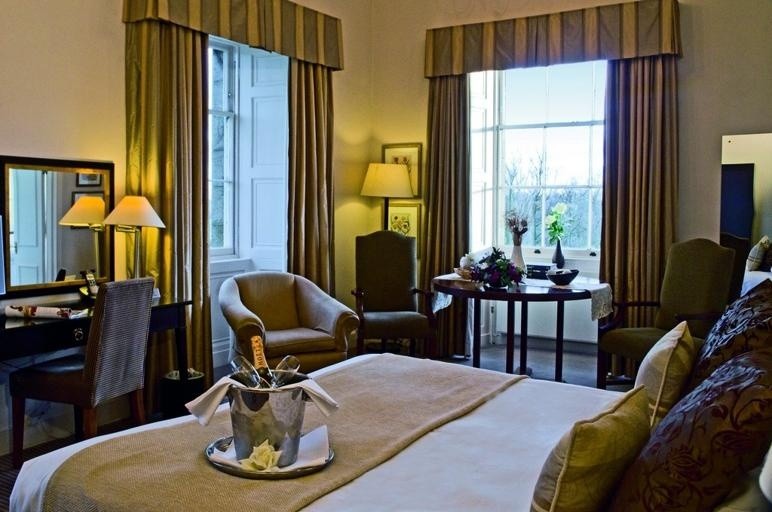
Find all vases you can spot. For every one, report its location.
[551,240,568,267]
[509,243,529,278]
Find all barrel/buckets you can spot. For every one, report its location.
[230,368,309,466]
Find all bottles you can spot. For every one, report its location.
[249,335,273,390]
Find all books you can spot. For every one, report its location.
[2,303,91,320]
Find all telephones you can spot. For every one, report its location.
[79,273,100,305]
[56,268,66,282]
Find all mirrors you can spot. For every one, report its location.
[0,155,118,300]
[720,131,772,273]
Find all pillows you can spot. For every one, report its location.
[619,347,771,512]
[692,276,772,385]
[530,382,652,512]
[631,316,697,416]
[746,229,771,270]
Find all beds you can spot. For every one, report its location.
[6,274,771,512]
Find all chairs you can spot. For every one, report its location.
[217,268,361,386]
[721,230,754,274]
[595,232,738,393]
[349,232,433,358]
[8,275,154,471]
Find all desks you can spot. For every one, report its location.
[425,264,612,382]
[0,294,194,418]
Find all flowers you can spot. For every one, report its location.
[542,201,572,243]
[504,207,533,244]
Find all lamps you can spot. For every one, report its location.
[102,192,168,277]
[361,160,415,232]
[57,195,109,279]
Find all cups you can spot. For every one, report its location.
[218,371,310,466]
[230,355,263,390]
[269,354,301,388]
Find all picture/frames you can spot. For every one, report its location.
[381,142,423,201]
[383,200,422,261]
[75,173,102,187]
[70,190,105,230]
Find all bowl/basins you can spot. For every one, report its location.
[547,270,580,285]
[453,267,473,279]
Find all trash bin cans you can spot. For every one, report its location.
[163,371,205,419]
